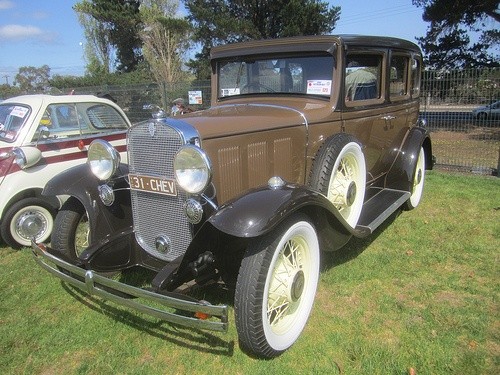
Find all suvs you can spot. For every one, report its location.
[32,34,437,359]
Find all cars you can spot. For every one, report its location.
[473,98,500,121]
[0,94,133,250]
